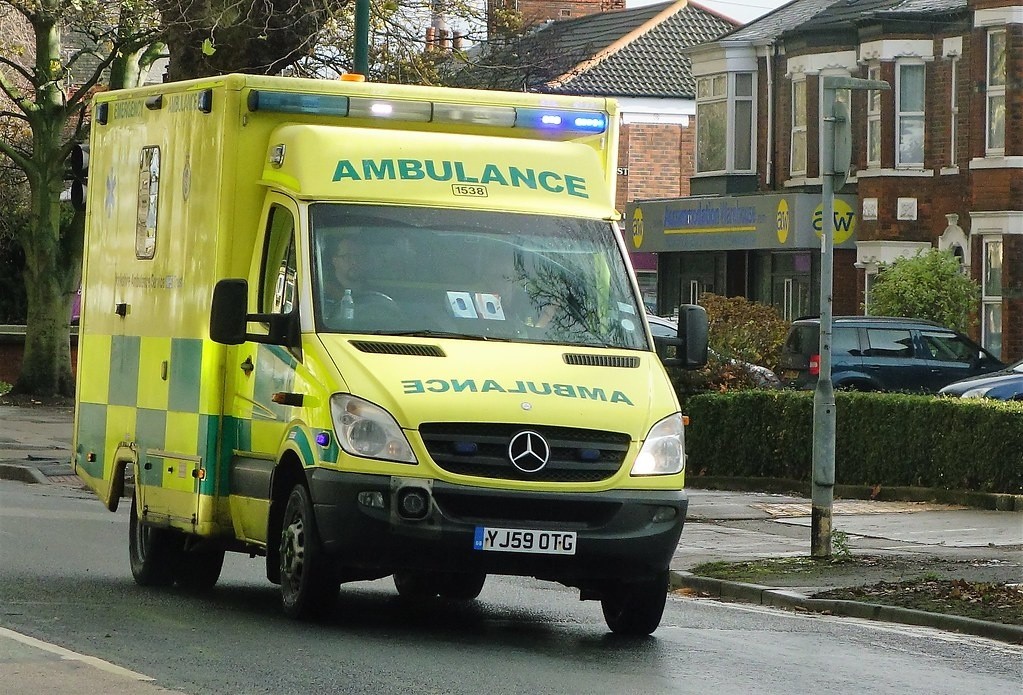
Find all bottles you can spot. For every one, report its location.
[341,289,354,318]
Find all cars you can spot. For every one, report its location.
[938,356,1023,404]
[614,302,780,389]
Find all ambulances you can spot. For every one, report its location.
[68,72,718,630]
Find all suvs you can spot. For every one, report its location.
[780,315,1011,395]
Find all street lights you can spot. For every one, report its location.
[809,72,896,558]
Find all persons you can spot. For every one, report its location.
[479,247,563,328]
[320,235,373,304]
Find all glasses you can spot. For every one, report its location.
[335,254,367,262]
